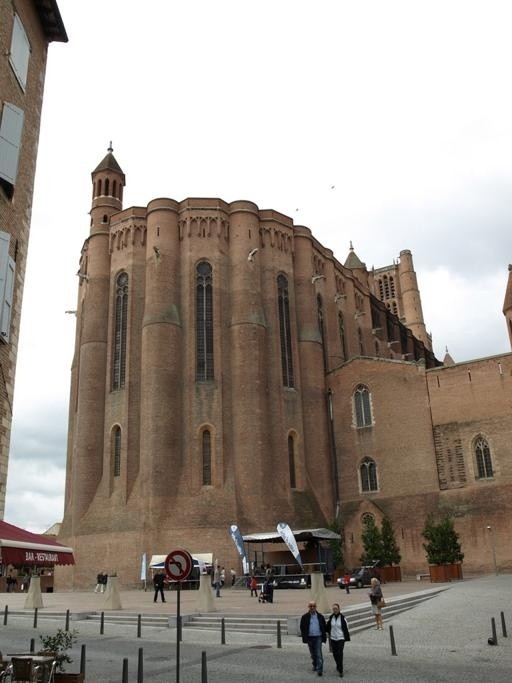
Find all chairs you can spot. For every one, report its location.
[10,656,40,683]
[54,643,86,683]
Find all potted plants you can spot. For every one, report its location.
[420,508,452,583]
[358,514,386,583]
[38,626,81,673]
[440,510,465,582]
[381,515,402,583]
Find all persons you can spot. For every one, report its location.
[326,604,351,677]
[214,560,273,602]
[94,570,104,592]
[344,572,351,594]
[300,602,327,676]
[368,578,384,630]
[8,568,16,591]
[103,572,108,591]
[23,573,30,593]
[153,569,166,603]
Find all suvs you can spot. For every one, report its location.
[337,566,381,588]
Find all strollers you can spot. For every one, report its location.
[259,581,270,601]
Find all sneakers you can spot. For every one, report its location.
[312,666,343,677]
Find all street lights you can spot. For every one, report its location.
[484,522,500,575]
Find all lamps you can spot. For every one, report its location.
[388,340,399,349]
[402,352,413,360]
[153,246,161,260]
[312,275,326,284]
[334,295,347,303]
[64,310,78,317]
[78,272,89,283]
[248,248,258,262]
[354,312,367,321]
[372,328,383,335]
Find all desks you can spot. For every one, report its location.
[167,579,200,591]
[3,654,55,683]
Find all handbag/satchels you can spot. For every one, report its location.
[376,600,387,608]
